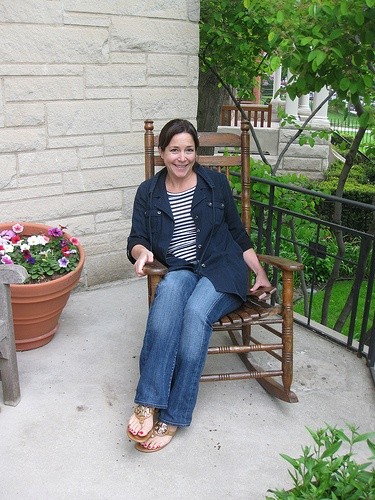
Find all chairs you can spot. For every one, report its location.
[0,264,29,408]
[143,118,304,404]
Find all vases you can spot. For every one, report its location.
[0,221,85,352]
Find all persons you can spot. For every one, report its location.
[127,119,271,453]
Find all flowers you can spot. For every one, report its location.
[0,224,81,278]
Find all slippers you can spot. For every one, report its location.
[136,420,177,453]
[127,404,160,442]
